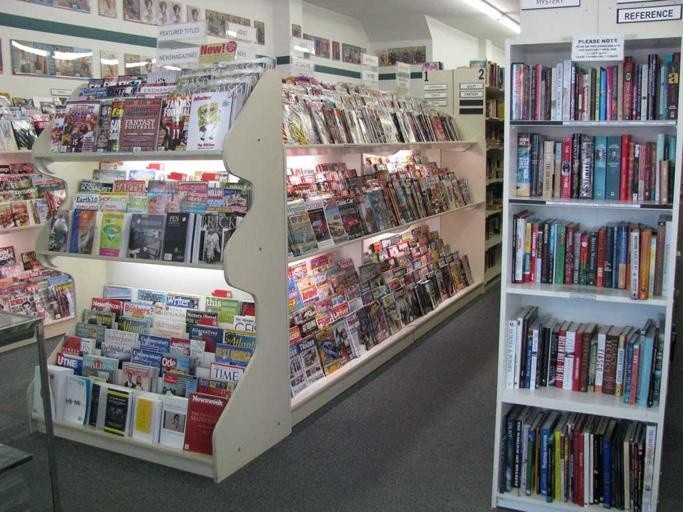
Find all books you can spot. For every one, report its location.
[501,54,682,122]
[503,304,666,408]
[504,405,657,512]
[517,132,676,206]
[1,46,503,456]
[511,210,672,301]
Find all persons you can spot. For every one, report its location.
[144,0,152,21]
[171,5,183,26]
[189,8,201,23]
[156,1,167,24]
